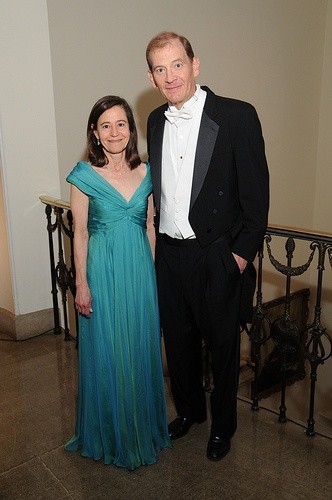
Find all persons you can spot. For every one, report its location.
[64,96,174,470]
[146,31,270,462]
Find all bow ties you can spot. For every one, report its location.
[164,111,192,124]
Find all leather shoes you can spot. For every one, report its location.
[168,415,208,440]
[207,431,230,461]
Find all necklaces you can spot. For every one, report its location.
[105,165,130,176]
